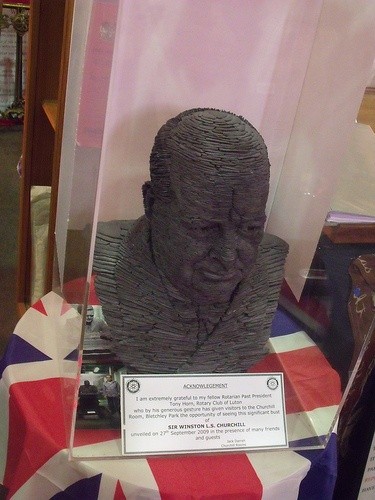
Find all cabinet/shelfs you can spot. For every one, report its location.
[14,0,76,321]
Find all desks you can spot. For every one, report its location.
[6,273,343,500]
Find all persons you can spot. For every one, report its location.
[91,102,287,377]
